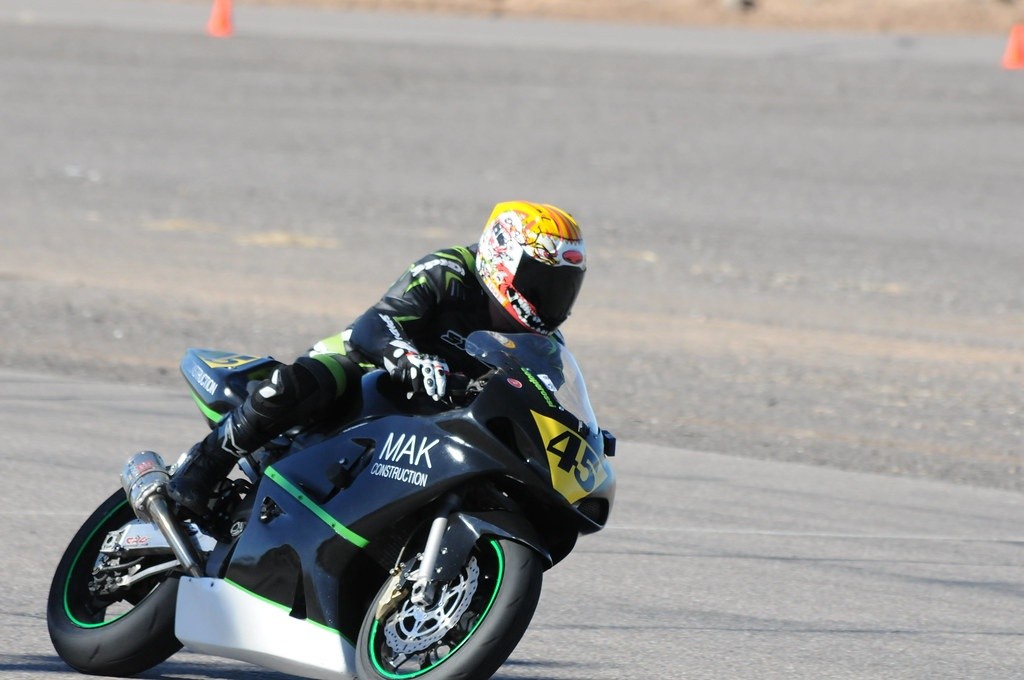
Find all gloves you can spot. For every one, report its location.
[383,340,449,401]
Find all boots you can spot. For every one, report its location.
[167,396,295,516]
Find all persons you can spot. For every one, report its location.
[163,200,590,515]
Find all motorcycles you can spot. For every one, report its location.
[46,333,622,675]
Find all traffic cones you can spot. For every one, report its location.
[1000,18,1024,73]
[204,1,235,37]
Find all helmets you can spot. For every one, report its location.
[474,199,587,338]
